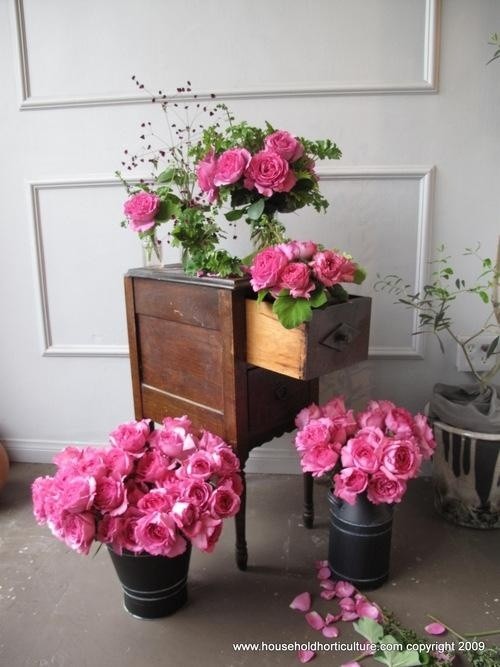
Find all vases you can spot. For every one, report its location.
[109,545,194,620]
[328,482,395,589]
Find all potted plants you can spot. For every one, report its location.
[376,243,498,530]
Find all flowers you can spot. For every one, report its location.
[108,76,368,330]
[292,394,433,507]
[29,413,244,559]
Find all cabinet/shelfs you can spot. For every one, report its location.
[125,265,372,574]
